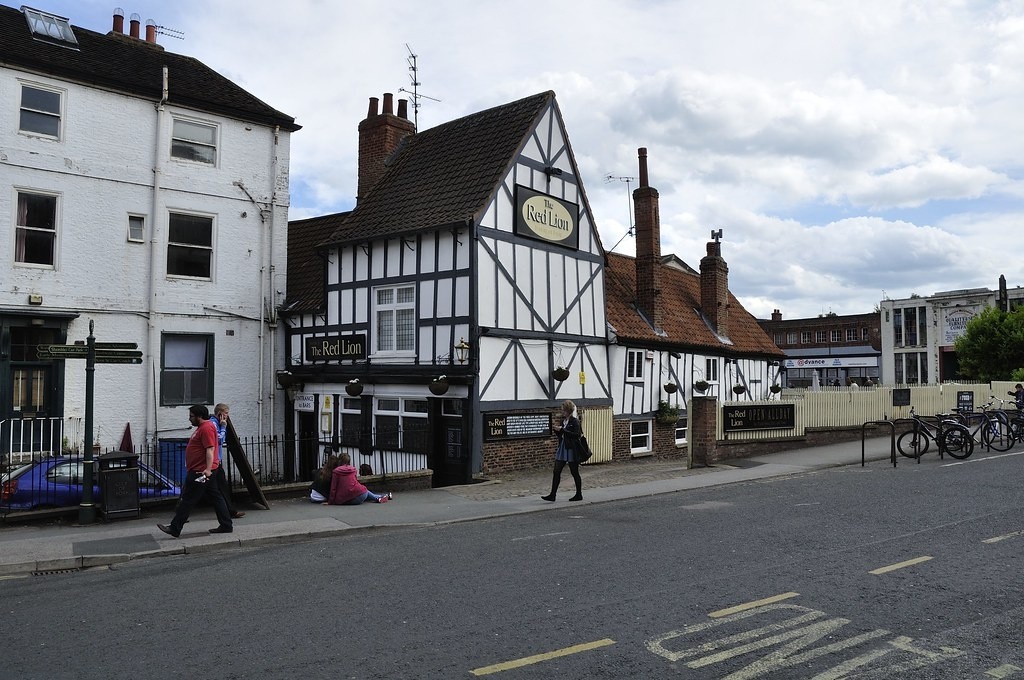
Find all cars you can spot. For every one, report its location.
[0,453,182,512]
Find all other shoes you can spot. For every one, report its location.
[157,524,179,537]
[569,495,582,501]
[232,512,245,518]
[389,493,392,500]
[209,525,233,533]
[379,496,388,503]
[541,494,555,501]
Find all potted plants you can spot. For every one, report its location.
[551,366,571,382]
[655,399,681,425]
[696,380,709,391]
[733,383,746,394]
[93,442,101,455]
[770,384,782,394]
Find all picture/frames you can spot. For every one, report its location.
[321,412,332,434]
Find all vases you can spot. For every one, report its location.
[345,385,363,397]
[428,383,450,396]
[278,375,293,384]
[664,385,678,394]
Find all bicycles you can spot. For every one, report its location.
[938,401,1015,452]
[897,405,974,460]
[989,395,1024,444]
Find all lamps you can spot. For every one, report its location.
[454,337,470,367]
[670,352,681,360]
[725,358,738,365]
[31,319,45,329]
[28,294,43,306]
[545,165,563,183]
[477,327,491,339]
[768,361,779,366]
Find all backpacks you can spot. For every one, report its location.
[570,418,592,462]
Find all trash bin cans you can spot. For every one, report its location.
[96,451,143,522]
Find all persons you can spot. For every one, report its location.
[541,399,583,502]
[849,379,859,387]
[1008,384,1024,409]
[320,453,393,505]
[834,379,841,386]
[863,376,873,387]
[819,382,824,386]
[306,454,339,504]
[157,405,233,537]
[185,403,246,523]
[788,384,795,388]
[845,377,852,386]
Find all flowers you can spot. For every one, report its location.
[664,380,676,386]
[431,375,448,384]
[348,378,361,386]
[276,371,293,376]
